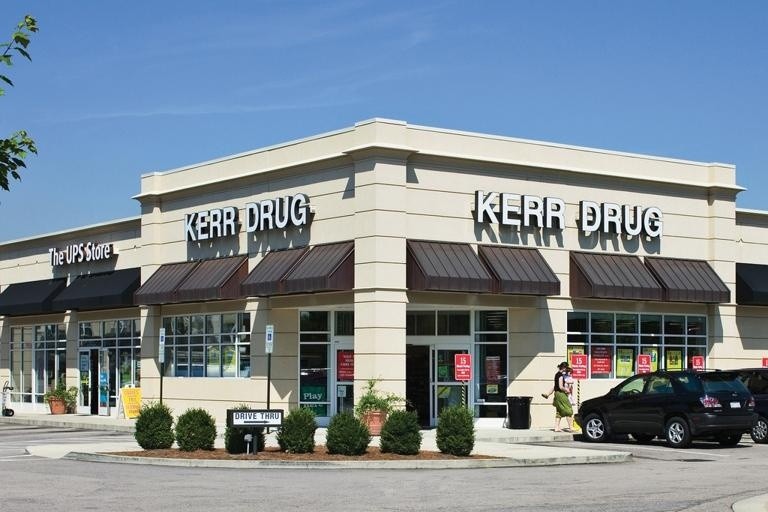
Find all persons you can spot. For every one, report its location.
[541,367,577,402]
[552,360,577,433]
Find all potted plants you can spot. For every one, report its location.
[353,379,414,436]
[44,378,77,414]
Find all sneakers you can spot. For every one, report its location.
[542,392,549,399]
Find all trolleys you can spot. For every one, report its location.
[1,380,14,417]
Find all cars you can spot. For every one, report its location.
[578,369,768,448]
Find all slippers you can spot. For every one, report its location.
[554,428,562,432]
[568,428,576,433]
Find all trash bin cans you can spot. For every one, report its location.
[505,396,533,429]
